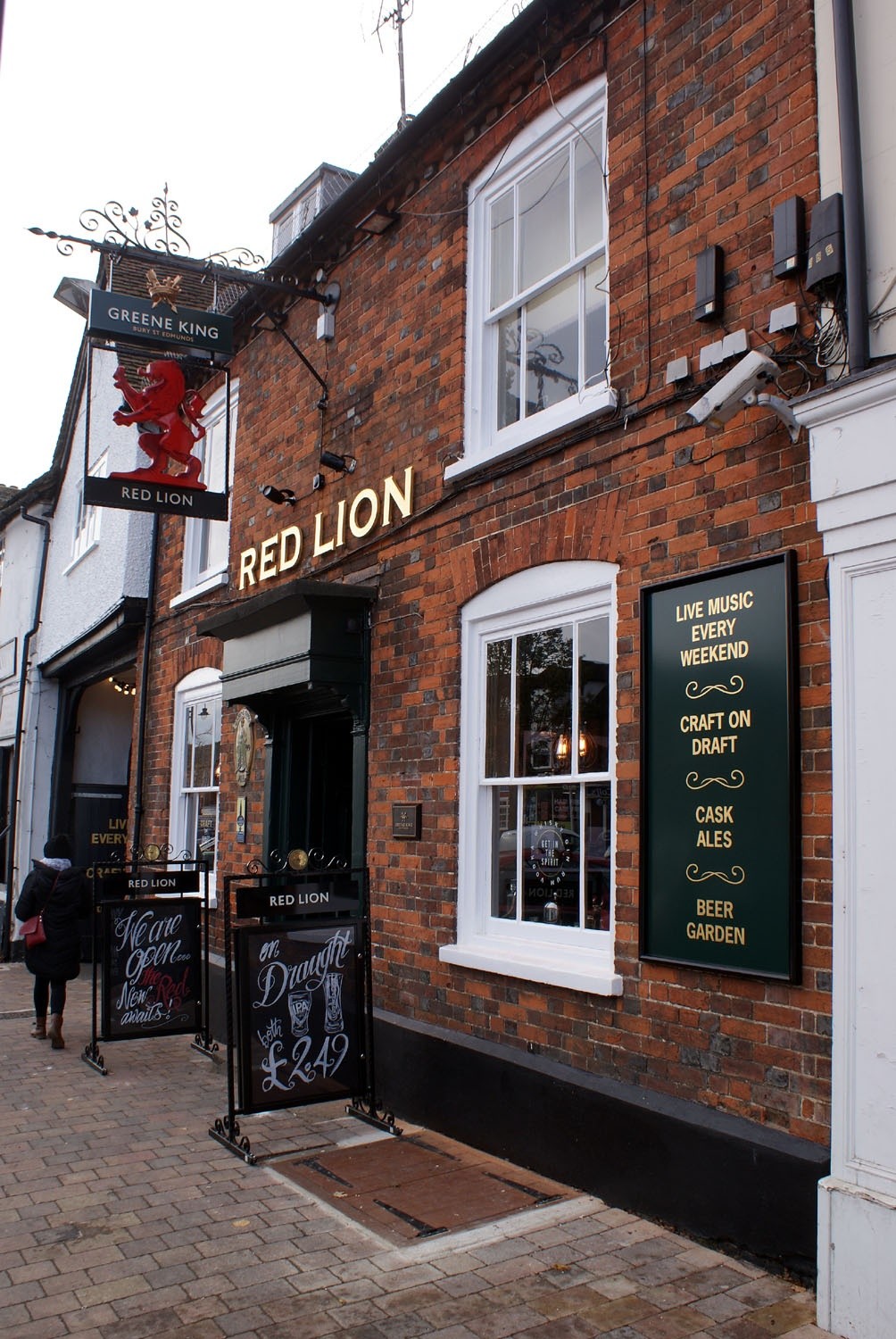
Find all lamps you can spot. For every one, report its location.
[320,452,356,473]
[109,676,137,697]
[355,209,399,235]
[263,485,296,507]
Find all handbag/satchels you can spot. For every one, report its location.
[18,917,46,947]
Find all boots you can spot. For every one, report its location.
[47,1014,66,1049]
[31,1018,47,1039]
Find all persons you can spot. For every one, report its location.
[15,834,87,1049]
